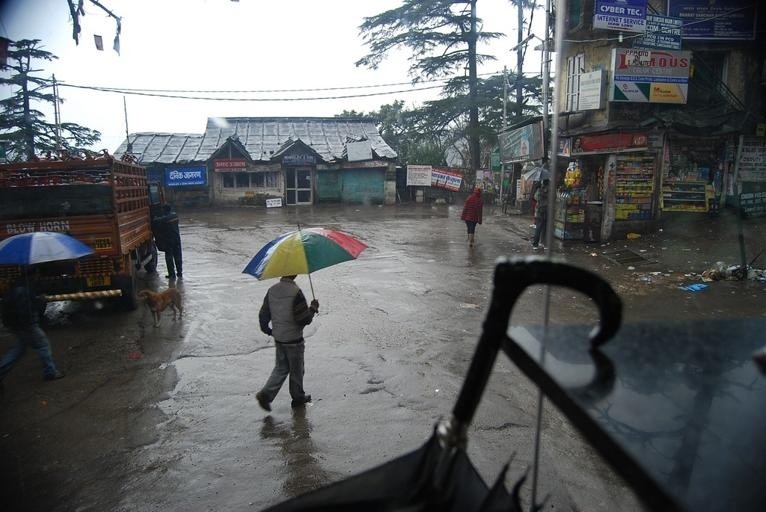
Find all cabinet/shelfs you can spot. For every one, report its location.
[552,183,587,241]
[595,152,722,220]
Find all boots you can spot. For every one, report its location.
[469,234,475,248]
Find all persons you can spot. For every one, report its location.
[461,187,484,248]
[529,179,550,251]
[254,276,319,412]
[0,265,66,381]
[572,137,584,153]
[152,204,182,278]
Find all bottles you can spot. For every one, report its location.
[616,191,632,203]
[559,192,573,206]
[683,176,687,182]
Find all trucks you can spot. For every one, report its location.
[0,148,156,315]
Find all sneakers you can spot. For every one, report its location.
[177,272,182,277]
[44,370,64,379]
[532,247,538,250]
[256,391,272,411]
[165,274,176,278]
[291,394,311,409]
[529,224,536,229]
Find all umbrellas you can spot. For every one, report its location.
[0,231,96,266]
[241,219,369,313]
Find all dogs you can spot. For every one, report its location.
[136,288,184,328]
